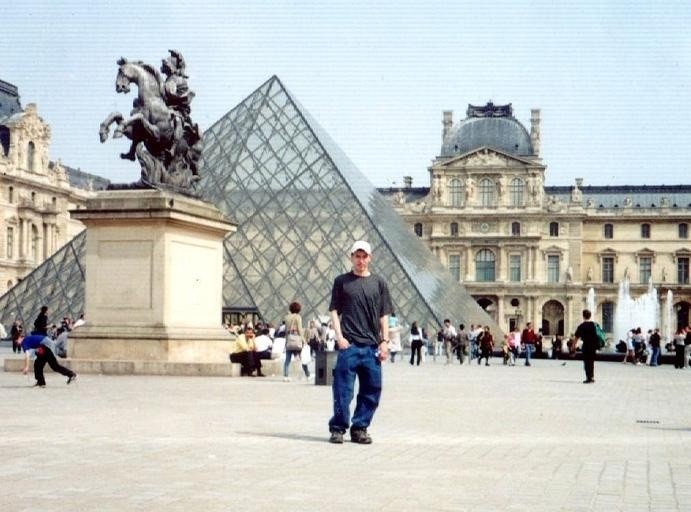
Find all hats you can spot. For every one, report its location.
[351,240,372,257]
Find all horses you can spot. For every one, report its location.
[97,56,202,180]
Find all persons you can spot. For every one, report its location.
[328,240,396,444]
[120,50,191,161]
[10,305,85,389]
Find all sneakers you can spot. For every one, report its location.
[33,384,46,389]
[350,426,371,444]
[329,431,344,443]
[67,373,78,384]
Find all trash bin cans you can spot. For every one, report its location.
[315,351,338,386]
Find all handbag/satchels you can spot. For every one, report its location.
[286,333,304,352]
[593,321,604,348]
